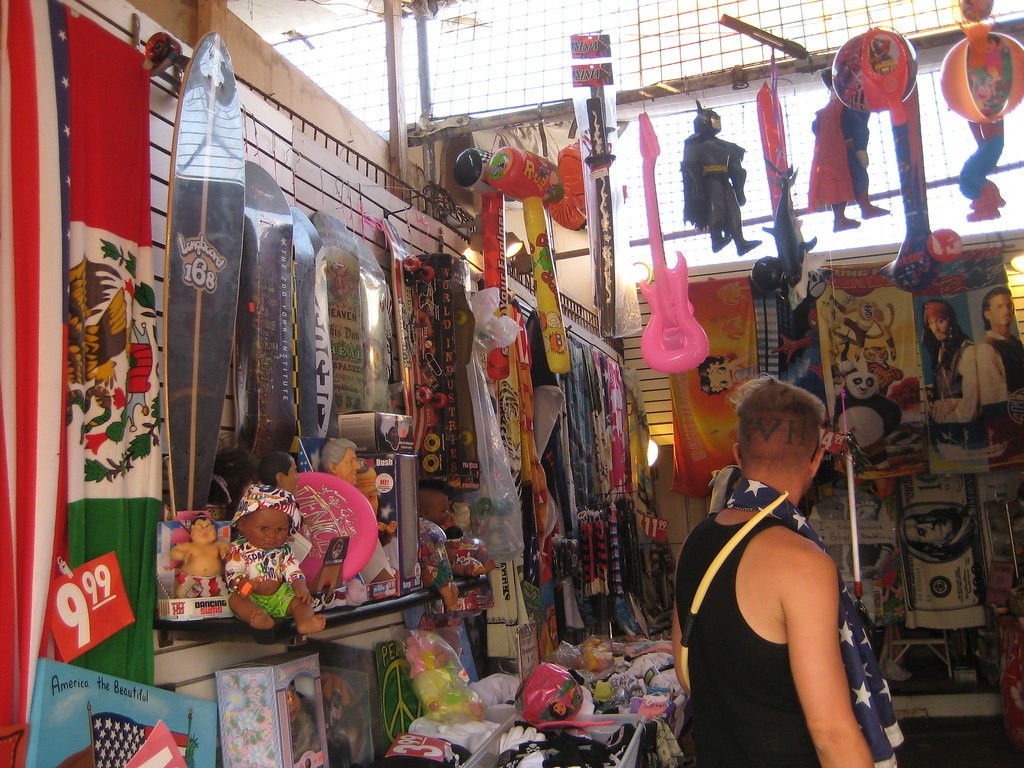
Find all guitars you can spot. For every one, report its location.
[637,110,710,376]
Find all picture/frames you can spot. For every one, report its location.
[287,638,385,768]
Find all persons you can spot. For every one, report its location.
[670,382,875,768]
[923,287,1024,446]
[170,437,360,634]
[417,487,495,612]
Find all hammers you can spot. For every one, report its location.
[456,144,573,378]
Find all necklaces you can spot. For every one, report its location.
[734,506,757,512]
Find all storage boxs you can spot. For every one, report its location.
[312,577,398,613]
[215,649,329,768]
[454,708,645,768]
[337,410,416,456]
[156,596,232,621]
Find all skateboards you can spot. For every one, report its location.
[230,158,393,474]
[382,214,449,456]
[138,29,249,523]
